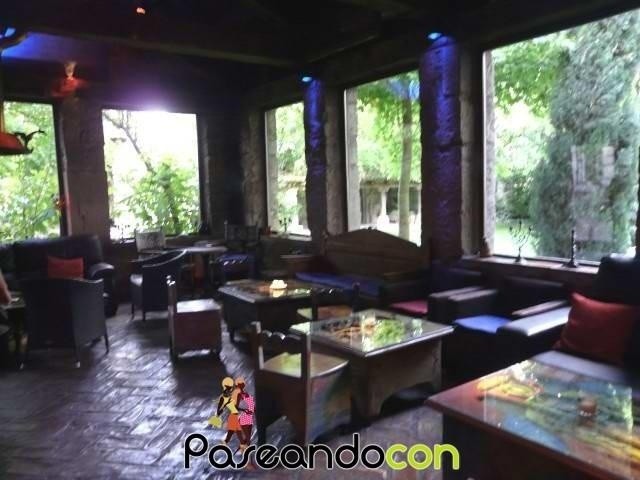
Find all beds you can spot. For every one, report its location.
[420,349,640,480]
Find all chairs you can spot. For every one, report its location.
[18,278,110,372]
[129,220,261,366]
[378,253,640,387]
[250,320,353,459]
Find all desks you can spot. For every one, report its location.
[217,278,454,427]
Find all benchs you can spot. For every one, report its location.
[280,226,432,312]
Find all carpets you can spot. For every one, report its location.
[252,404,444,480]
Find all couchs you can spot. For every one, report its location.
[0,233,121,317]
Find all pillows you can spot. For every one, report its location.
[46,255,85,280]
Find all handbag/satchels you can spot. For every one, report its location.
[239,399,249,410]
[207,415,222,429]
